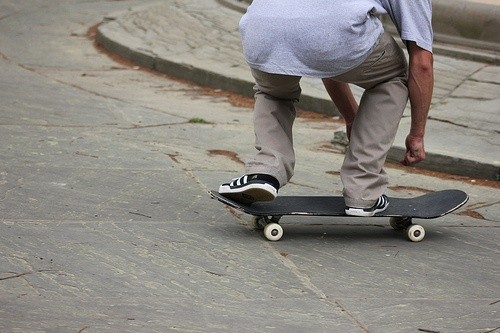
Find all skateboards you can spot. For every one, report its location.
[206,189,470,242]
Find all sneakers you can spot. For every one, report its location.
[218,174,280,207]
[345,194,390,216]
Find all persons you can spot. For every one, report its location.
[219,0,432,215]
